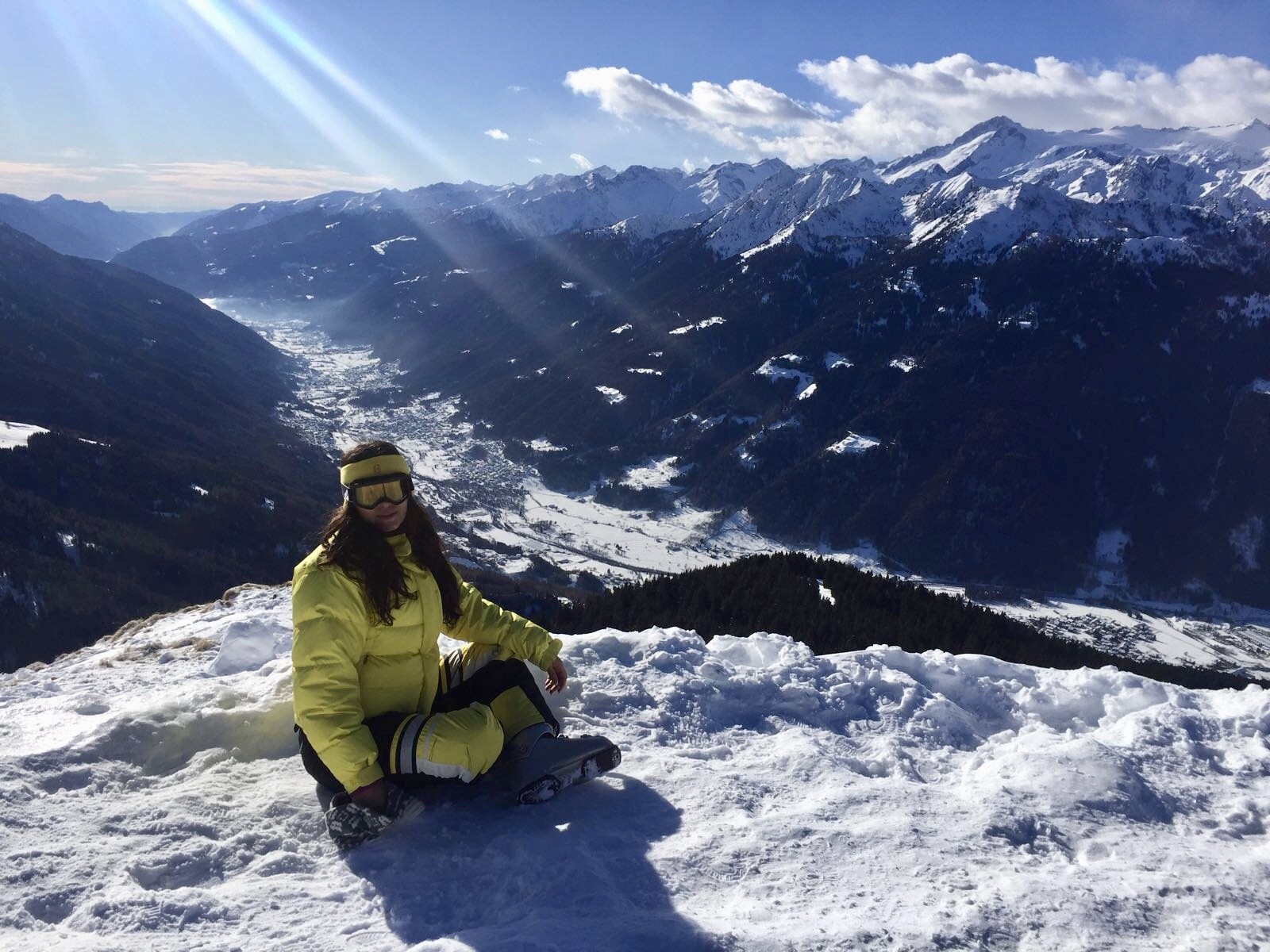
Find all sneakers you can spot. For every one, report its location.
[509,733,621,804]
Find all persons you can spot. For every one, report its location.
[292,440,621,840]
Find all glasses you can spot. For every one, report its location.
[350,474,408,509]
